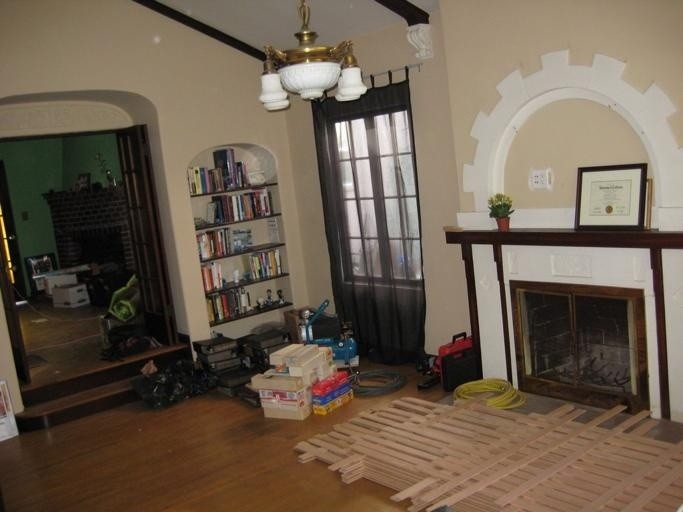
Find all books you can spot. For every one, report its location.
[188,148,282,324]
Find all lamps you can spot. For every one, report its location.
[256,2,368,112]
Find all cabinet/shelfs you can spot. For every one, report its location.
[189,182,293,327]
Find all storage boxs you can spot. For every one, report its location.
[44,273,106,311]
[192,302,363,422]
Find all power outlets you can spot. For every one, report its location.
[532,170,547,190]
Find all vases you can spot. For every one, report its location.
[495,216,511,232]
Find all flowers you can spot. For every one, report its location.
[486,193,517,220]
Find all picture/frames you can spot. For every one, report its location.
[644,178,654,230]
[574,161,648,231]
[78,173,91,194]
[24,252,60,300]
